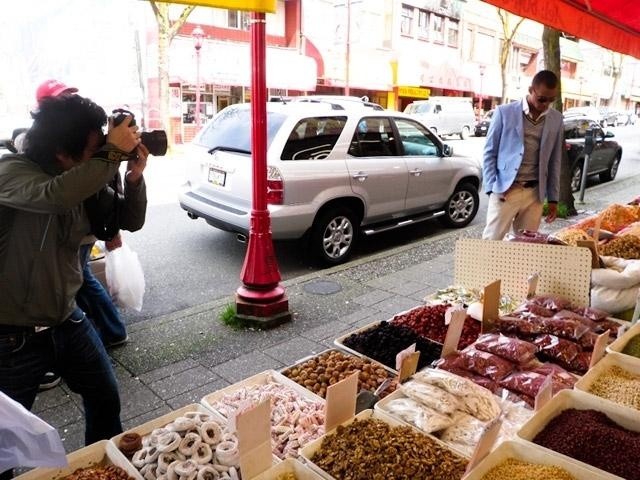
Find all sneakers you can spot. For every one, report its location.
[37,373,60,391]
[99,330,129,348]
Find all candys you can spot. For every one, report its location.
[210,373,326,460]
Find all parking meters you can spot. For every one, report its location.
[579,129,596,207]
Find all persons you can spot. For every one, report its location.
[13,76,131,390]
[481,69,564,241]
[0,93,149,480]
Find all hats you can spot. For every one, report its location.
[36,77,79,103]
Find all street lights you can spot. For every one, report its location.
[626,78,637,110]
[478,61,493,124]
[190,22,204,135]
[578,76,585,108]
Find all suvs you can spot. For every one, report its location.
[176,101,484,267]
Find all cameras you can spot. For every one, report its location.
[109,109,167,160]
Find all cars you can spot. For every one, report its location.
[564,103,638,127]
[1,240,106,355]
[279,92,384,104]
[475,108,496,138]
[560,111,624,191]
[0,91,34,155]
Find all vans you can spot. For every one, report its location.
[399,95,477,139]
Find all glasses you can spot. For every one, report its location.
[533,89,558,103]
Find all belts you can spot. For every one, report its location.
[1,324,49,335]
[523,180,538,188]
